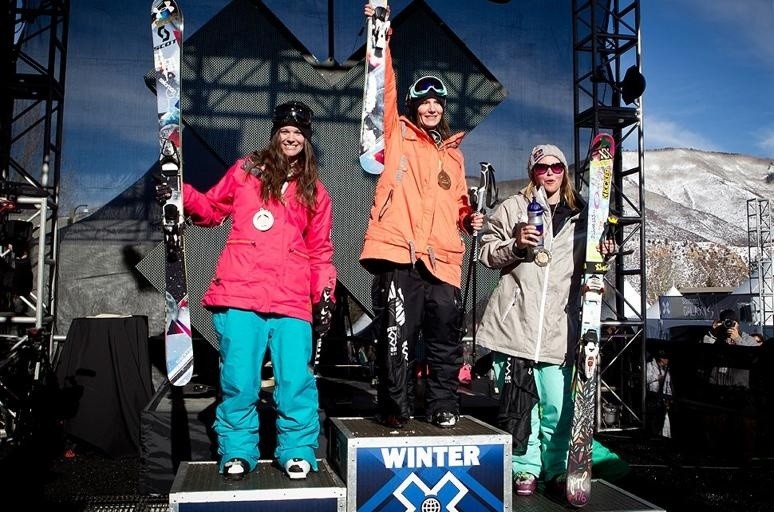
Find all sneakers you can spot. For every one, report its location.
[553,472,567,491]
[513,471,537,496]
[224,458,249,481]
[284,458,310,479]
[385,415,403,427]
[428,408,457,428]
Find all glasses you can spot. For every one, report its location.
[272,104,313,127]
[533,163,563,175]
[405,76,448,107]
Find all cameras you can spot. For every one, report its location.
[722,319,734,328]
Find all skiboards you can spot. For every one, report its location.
[152,0,195,388]
[359,0,388,175]
[567,134,614,508]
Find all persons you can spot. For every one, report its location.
[360,3,483,427]
[155,100,337,480]
[701,307,760,387]
[601,317,635,335]
[646,354,673,438]
[475,143,618,497]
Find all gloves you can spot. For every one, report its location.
[315,300,336,332]
[151,173,172,207]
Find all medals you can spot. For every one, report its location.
[438,170,451,190]
[535,248,552,267]
[252,210,274,231]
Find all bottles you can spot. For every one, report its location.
[527,196,544,250]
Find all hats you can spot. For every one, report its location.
[409,91,445,119]
[269,101,312,144]
[720,309,739,324]
[528,144,569,184]
